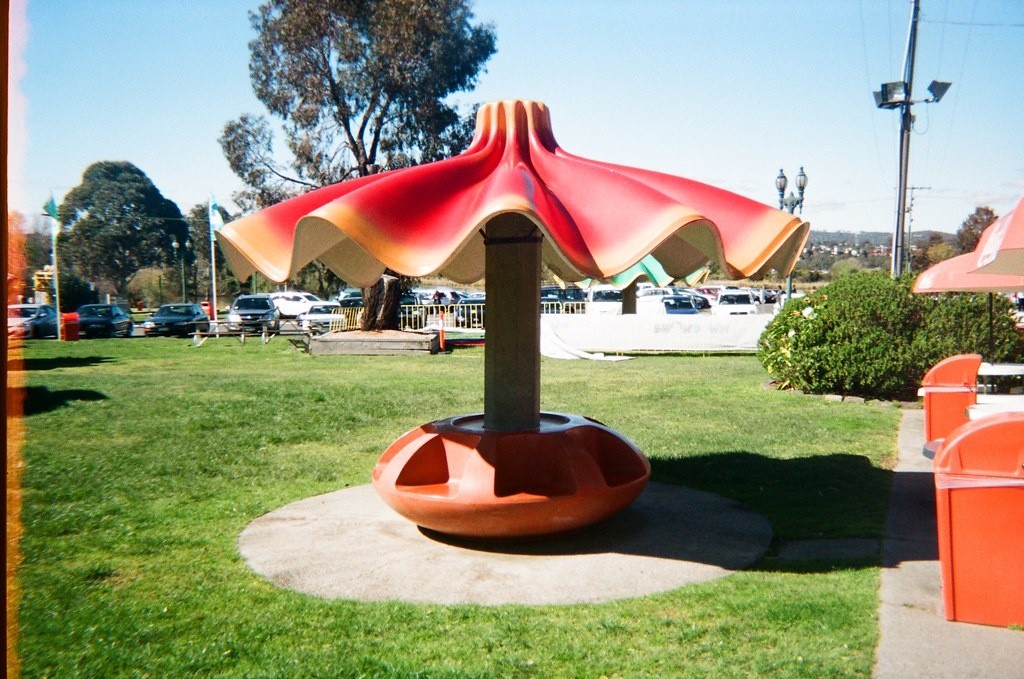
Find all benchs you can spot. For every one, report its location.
[257,319,323,345]
[187,321,251,347]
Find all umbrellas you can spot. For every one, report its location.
[553,253,709,318]
[209,95,811,428]
[913,197,1023,394]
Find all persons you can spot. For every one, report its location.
[759,284,820,304]
[433,291,441,306]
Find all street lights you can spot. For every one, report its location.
[172,239,193,304]
[776,165,809,302]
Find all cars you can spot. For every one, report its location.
[226,294,280,336]
[73,304,134,339]
[336,286,777,314]
[397,295,428,328]
[141,303,210,336]
[6,304,59,339]
[271,291,343,316]
[710,289,757,316]
[663,294,700,315]
[455,298,485,327]
[777,288,808,310]
[299,300,346,335]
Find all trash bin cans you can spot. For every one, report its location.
[916,354,984,442]
[200,301,213,320]
[922,412,1024,629]
